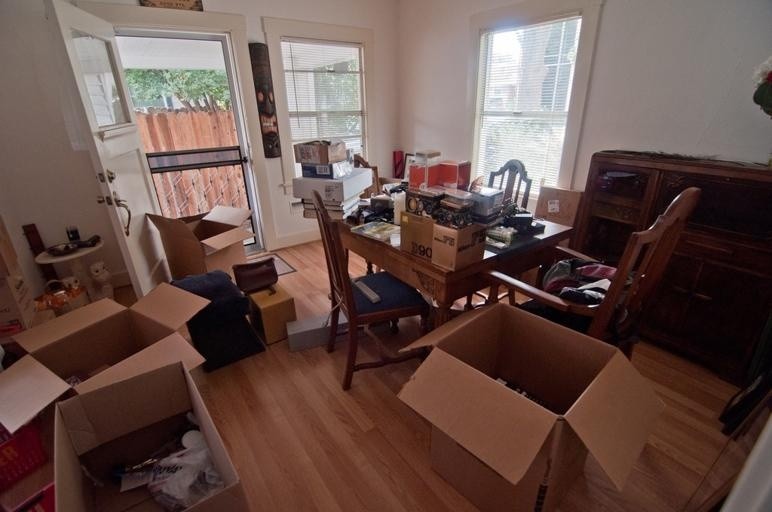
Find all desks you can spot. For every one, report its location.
[35,240,106,304]
[332,205,579,373]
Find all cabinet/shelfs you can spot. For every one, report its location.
[576,152,661,269]
[635,155,770,385]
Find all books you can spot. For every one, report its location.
[350,220,400,242]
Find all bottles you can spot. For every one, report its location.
[394,188,406,225]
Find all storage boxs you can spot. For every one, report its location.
[292,139,503,268]
[1,283,210,434]
[535,185,583,229]
[251,285,297,345]
[0,222,57,367]
[390,301,666,512]
[145,204,256,286]
[54,360,250,511]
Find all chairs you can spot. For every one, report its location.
[489,181,704,369]
[488,156,530,204]
[303,188,431,394]
